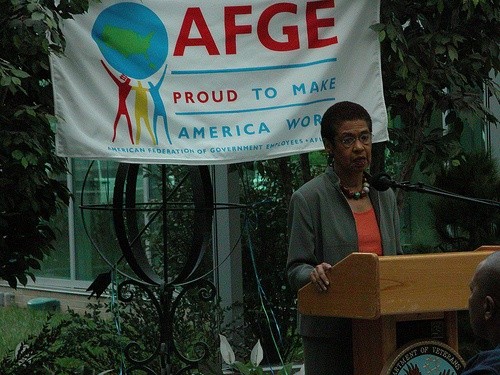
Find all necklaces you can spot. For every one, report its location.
[338,177,377,199]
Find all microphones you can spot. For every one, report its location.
[372,173,407,192]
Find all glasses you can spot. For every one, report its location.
[335,133,374,149]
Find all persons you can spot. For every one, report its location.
[286,101,403,375]
[454,250,500,375]
